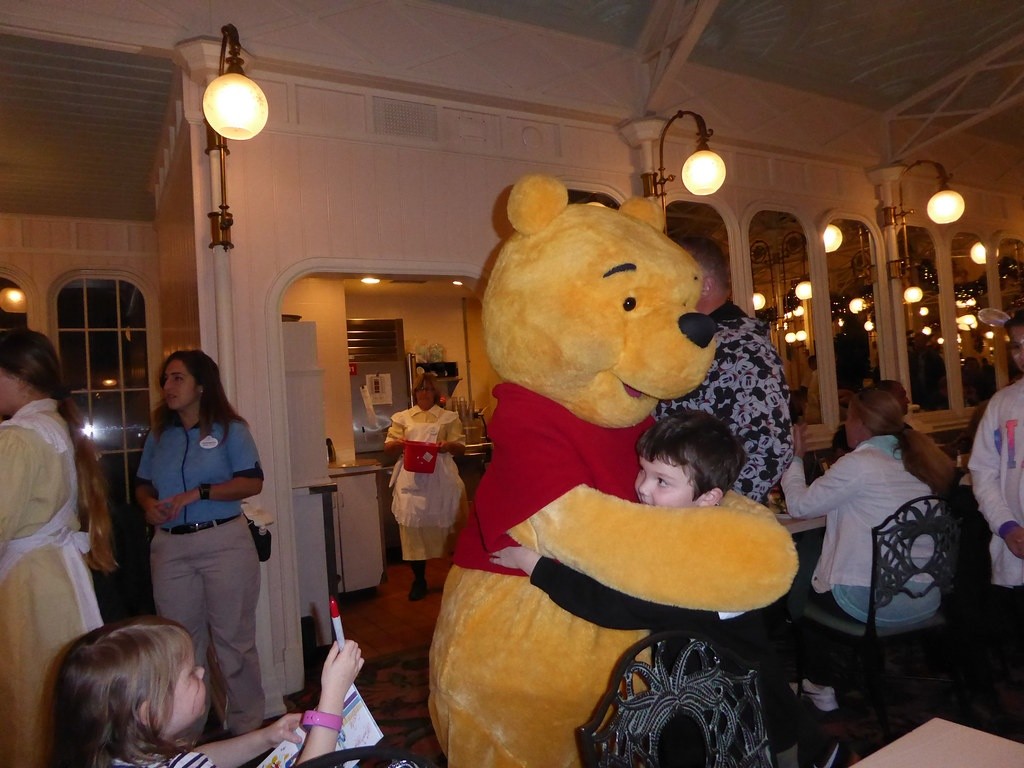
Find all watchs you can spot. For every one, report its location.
[200,482,211,501]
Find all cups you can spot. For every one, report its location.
[452,397,475,418]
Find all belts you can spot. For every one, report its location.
[160,514,241,535]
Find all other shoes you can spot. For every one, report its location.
[409,578,427,601]
[790,679,839,711]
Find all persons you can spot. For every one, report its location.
[383,373,470,600]
[802,331,997,424]
[649,235,795,505]
[49,613,365,768]
[779,379,956,713]
[0,328,123,766]
[136,350,264,737]
[486,411,807,767]
[968,310,1024,699]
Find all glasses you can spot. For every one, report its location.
[419,385,433,391]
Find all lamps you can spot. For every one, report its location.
[0,282,29,321]
[904,152,969,228]
[179,23,276,249]
[639,102,730,201]
[744,220,1016,370]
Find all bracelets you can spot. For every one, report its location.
[197,485,203,500]
[999,521,1019,539]
[302,710,343,734]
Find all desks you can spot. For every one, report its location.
[765,496,826,538]
[840,716,1024,768]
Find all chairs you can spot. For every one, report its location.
[575,624,779,768]
[789,491,966,709]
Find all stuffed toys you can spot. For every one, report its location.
[426,176,800,767]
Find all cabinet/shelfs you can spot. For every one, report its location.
[327,460,387,599]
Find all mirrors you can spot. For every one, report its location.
[735,199,833,444]
[941,222,1005,417]
[885,216,963,421]
[815,208,899,433]
[986,228,1024,390]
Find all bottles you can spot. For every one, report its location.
[259,526,266,535]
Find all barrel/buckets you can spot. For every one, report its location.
[403,441,438,473]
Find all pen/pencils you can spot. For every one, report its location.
[329,596,346,651]
